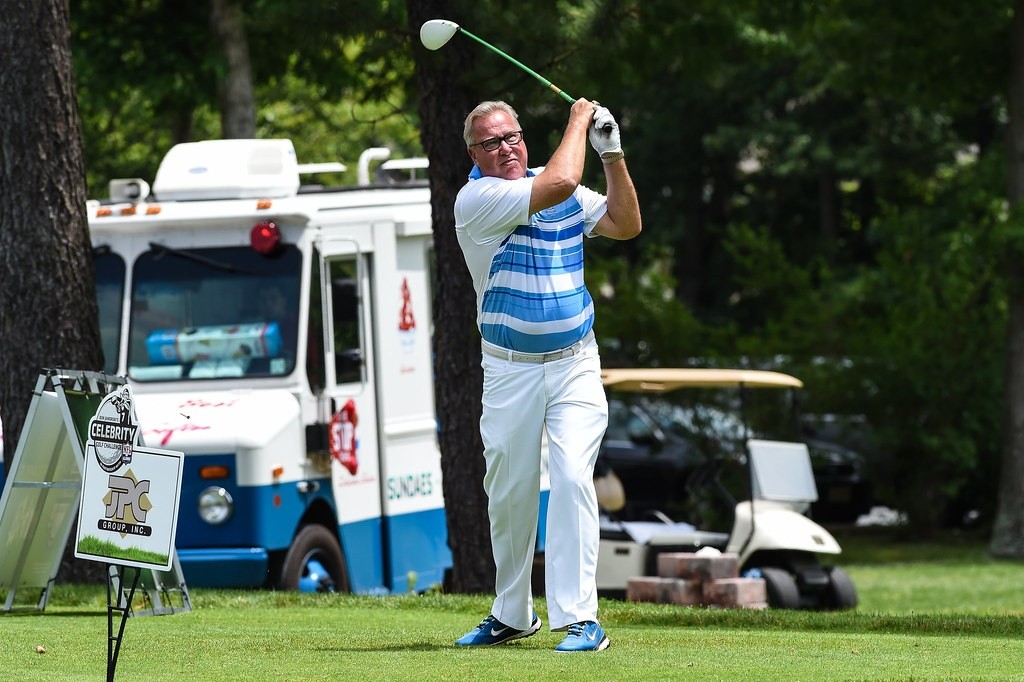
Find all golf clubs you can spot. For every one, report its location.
[419,18,614,136]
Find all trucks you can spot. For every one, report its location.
[85,137,555,598]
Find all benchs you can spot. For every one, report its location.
[610,500,728,548]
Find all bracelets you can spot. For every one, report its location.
[601,149,625,163]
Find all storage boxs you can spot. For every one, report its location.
[145,321,283,364]
[625,551,770,611]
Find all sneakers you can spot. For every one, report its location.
[454,611,542,648]
[556,621,610,652]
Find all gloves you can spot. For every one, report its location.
[588,100,621,157]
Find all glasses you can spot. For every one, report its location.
[470,131,525,152]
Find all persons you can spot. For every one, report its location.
[454,98,642,651]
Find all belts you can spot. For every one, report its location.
[481,326,595,363]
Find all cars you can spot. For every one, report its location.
[594,396,874,536]
